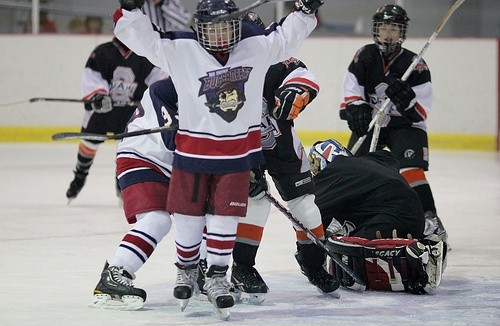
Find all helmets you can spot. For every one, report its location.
[308,139,352,175]
[243,11,265,29]
[190,0,243,52]
[113,9,123,25]
[372,4,409,52]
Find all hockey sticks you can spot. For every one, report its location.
[247,175,367,293]
[211,0,272,25]
[28,97,141,106]
[349,0,466,155]
[52,123,179,141]
[368,110,387,153]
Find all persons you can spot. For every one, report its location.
[87,76,243,302]
[338,4,452,252]
[113,0,324,308]
[230,12,340,294]
[65,35,153,199]
[308,139,447,294]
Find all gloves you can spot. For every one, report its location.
[272,85,310,121]
[119,0,144,11]
[386,73,415,111]
[91,94,112,112]
[295,0,323,14]
[247,168,268,199]
[346,101,373,137]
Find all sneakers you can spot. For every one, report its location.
[423,210,448,240]
[202,265,235,321]
[174,262,198,311]
[88,261,147,311]
[296,252,340,298]
[230,263,268,304]
[66,171,88,204]
